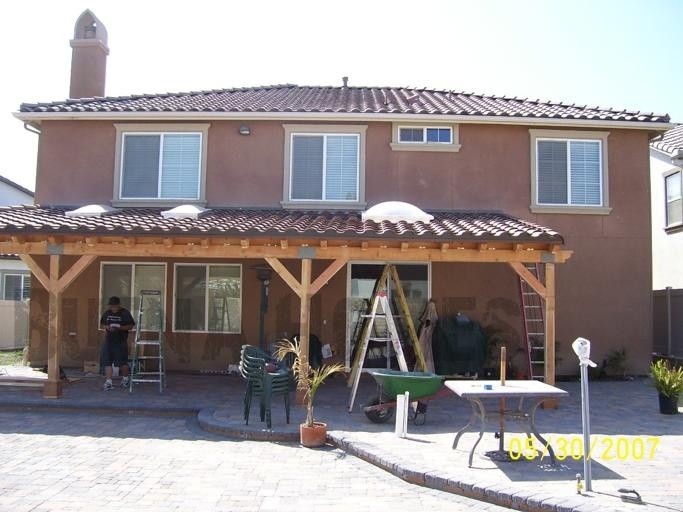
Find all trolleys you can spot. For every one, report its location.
[363,368,451,425]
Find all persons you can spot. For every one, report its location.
[99,297,135,391]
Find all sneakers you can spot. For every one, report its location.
[101,382,114,391]
[120,378,130,388]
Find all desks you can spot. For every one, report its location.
[444,379,569,468]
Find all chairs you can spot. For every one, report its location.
[237,343,292,429]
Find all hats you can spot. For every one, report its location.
[107,296,120,305]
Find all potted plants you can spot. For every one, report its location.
[271,335,349,449]
[642,359,683,414]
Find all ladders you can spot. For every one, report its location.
[346,264,428,389]
[517,261,547,382]
[128,289,167,396]
[346,285,420,418]
